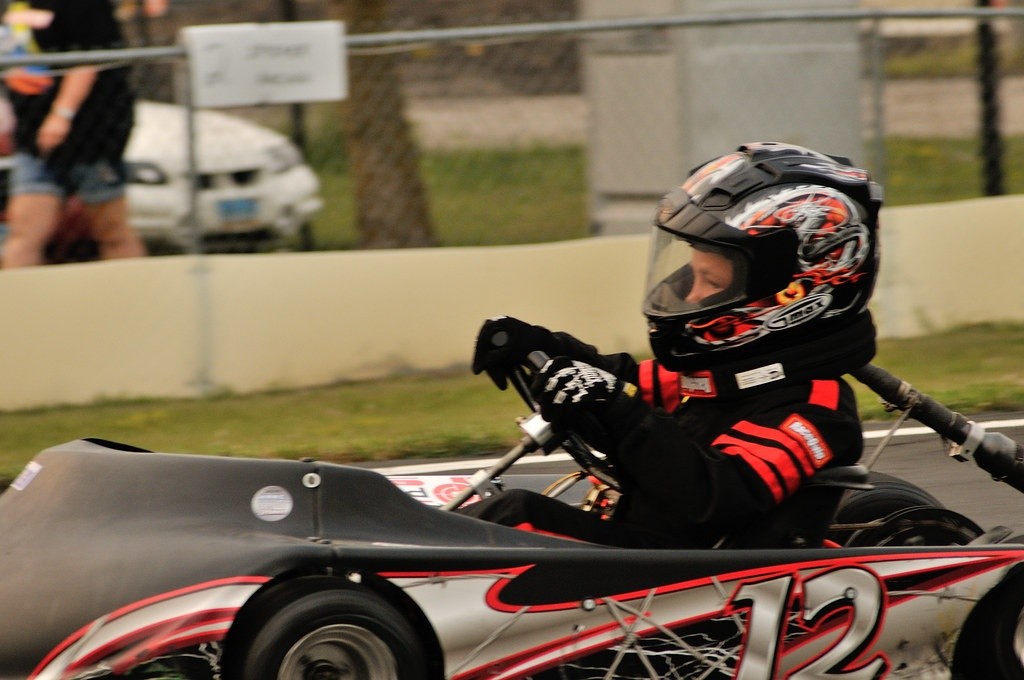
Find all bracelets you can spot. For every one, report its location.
[49,105,75,121]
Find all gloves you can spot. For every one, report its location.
[472,316,598,390]
[534,356,640,434]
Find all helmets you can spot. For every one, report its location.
[643,141,884,381]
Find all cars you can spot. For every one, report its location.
[1,89,326,256]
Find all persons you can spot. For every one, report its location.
[0,0,136,272]
[456,140,883,551]
